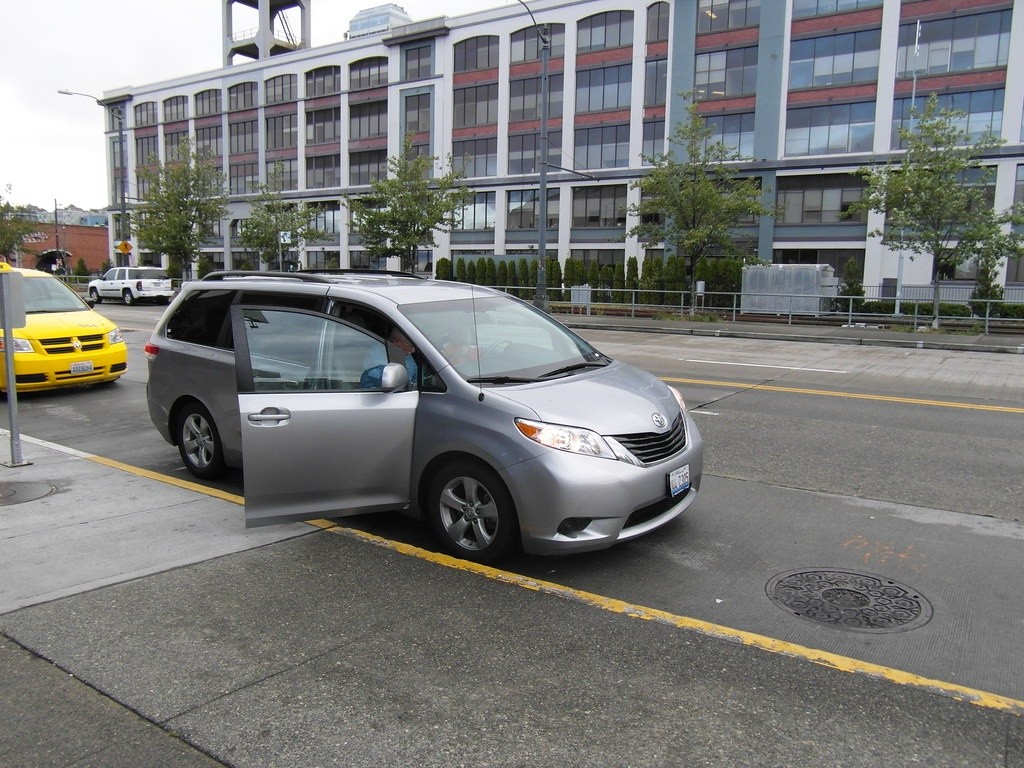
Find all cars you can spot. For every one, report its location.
[0,268,129,396]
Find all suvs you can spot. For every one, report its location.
[146,269,703,567]
[88,266,175,307]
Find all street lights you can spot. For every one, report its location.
[57,89,130,267]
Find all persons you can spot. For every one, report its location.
[441,320,485,374]
[359,326,417,390]
